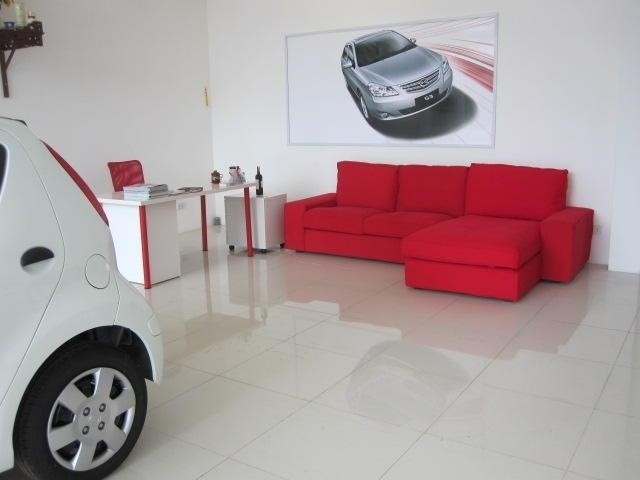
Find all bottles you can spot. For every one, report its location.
[254,164,264,196]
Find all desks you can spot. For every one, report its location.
[96,182,258,289]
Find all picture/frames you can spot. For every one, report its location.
[284,10,500,149]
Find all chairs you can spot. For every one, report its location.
[107,160,146,191]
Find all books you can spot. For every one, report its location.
[178,185,203,193]
[123,181,168,198]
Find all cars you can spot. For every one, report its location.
[340,30,455,126]
[0,115,168,479]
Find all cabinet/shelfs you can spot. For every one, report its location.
[224,192,287,253]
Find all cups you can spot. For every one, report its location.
[210,170,224,183]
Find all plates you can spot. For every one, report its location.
[209,181,223,185]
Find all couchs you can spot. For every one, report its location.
[284,159,595,304]
